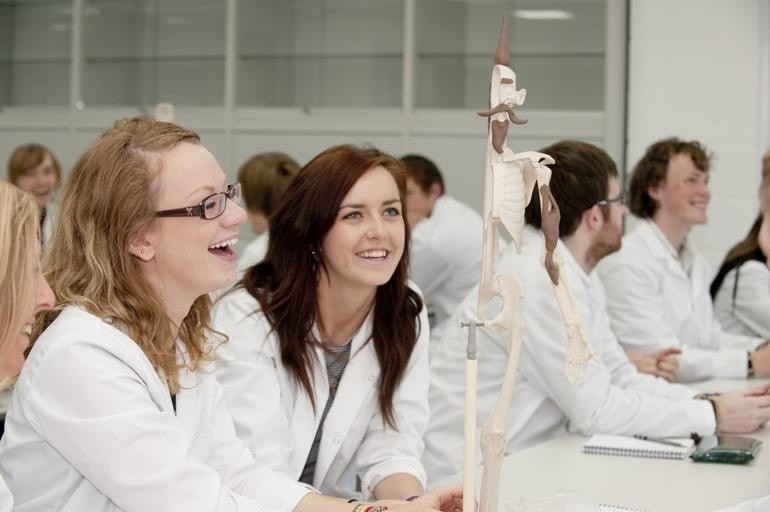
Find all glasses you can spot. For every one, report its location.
[149,181,243,220]
[599,192,627,206]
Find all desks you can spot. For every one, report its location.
[425,432,770,512]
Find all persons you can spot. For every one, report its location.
[0,112,770,512]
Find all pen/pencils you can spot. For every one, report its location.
[632,433,686,449]
